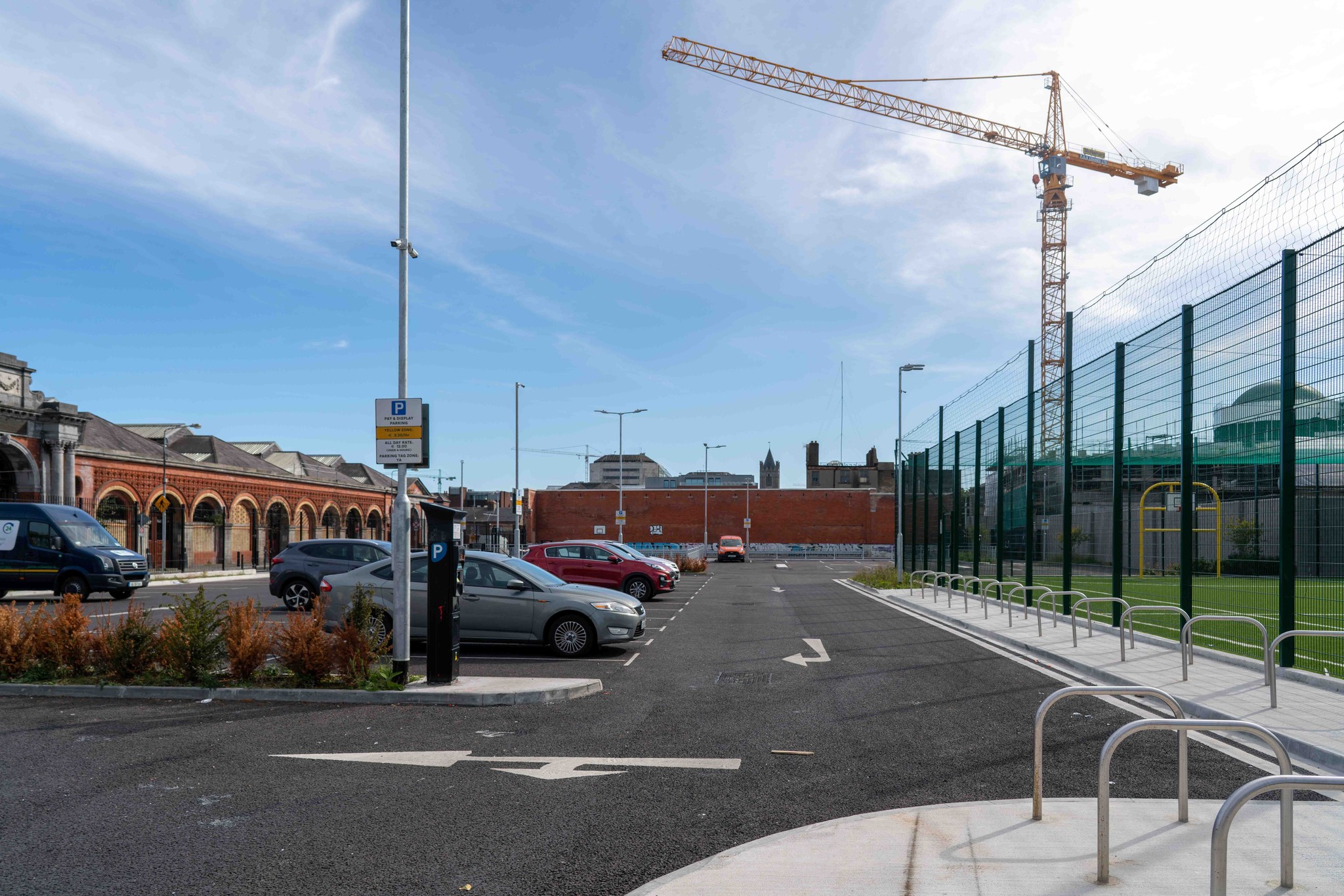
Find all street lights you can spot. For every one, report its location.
[514,381,525,558]
[162,424,202,572]
[595,409,647,544]
[745,477,752,559]
[704,443,727,559]
[897,363,925,583]
[390,468,418,542]
[460,459,465,546]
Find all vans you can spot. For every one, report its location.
[716,535,747,562]
[0,502,150,603]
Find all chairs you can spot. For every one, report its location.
[480,567,494,587]
[464,567,479,586]
[360,548,370,562]
[585,548,594,560]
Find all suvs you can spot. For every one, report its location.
[520,542,676,602]
[269,538,412,612]
[557,540,681,583]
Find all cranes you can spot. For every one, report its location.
[659,33,1186,485]
[381,469,456,494]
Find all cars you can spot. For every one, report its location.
[310,550,647,658]
